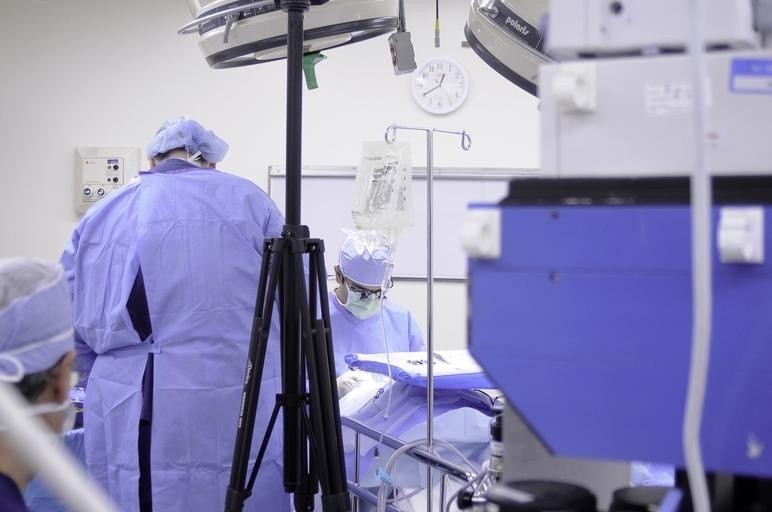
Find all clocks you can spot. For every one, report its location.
[412,57,467,115]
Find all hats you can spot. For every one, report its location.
[145,116,229,164]
[337,227,394,288]
[0,255,75,380]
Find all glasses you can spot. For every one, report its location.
[342,279,388,300]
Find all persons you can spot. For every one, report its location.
[306,230,426,400]
[57,118,323,512]
[0,257,77,512]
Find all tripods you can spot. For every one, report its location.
[225,225,352,512]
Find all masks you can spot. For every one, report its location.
[340,283,383,321]
